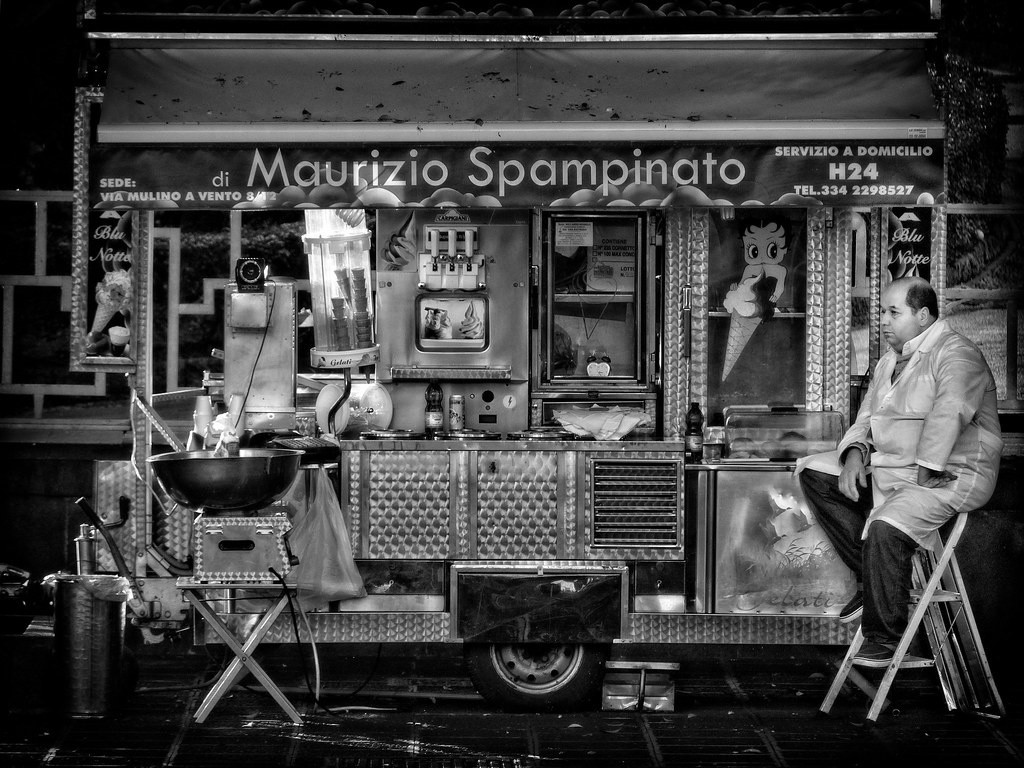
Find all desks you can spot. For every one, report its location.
[176,579,302,724]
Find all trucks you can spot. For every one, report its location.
[0,12,1023,709]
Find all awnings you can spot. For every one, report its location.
[93,47,945,210]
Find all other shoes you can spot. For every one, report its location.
[839,590,864,623]
[852,643,910,667]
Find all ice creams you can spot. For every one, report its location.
[379,210,418,271]
[423,306,453,340]
[91,268,131,332]
[457,300,485,340]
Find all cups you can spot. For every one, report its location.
[109,326,130,356]
[228,394,246,439]
[193,395,213,439]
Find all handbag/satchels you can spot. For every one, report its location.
[281,464,368,613]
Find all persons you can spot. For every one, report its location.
[793,278,1004,668]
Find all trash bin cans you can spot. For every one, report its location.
[44,572,134,722]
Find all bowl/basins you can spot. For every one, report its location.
[145,448,306,515]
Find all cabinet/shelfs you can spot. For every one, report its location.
[665,205,839,448]
[531,208,654,435]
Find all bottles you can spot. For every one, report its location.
[685,402,704,460]
[425,378,443,439]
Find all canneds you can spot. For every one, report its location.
[449,395,465,431]
